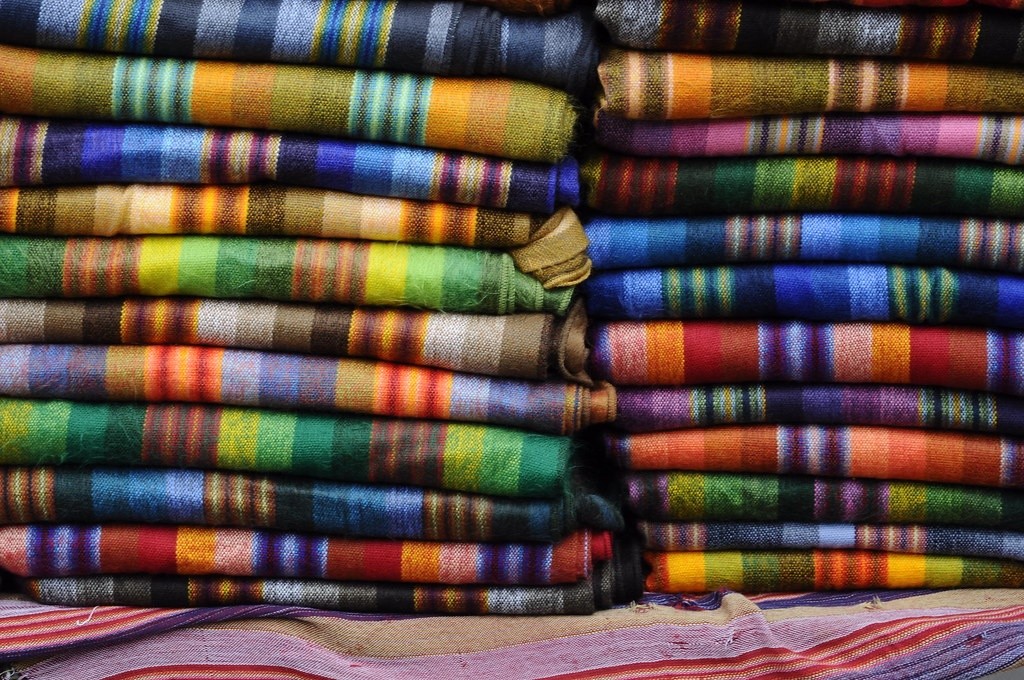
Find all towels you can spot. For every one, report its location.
[0,0,1024,674]
